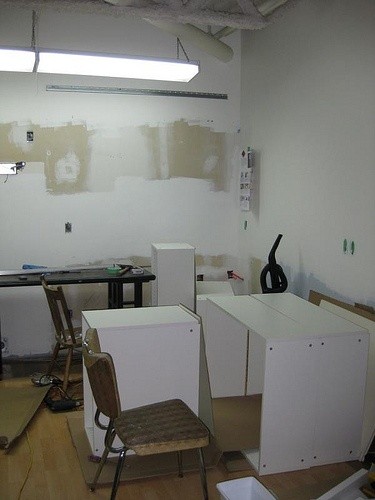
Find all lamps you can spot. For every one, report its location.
[0,10,201,83]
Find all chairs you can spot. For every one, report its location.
[40,273,88,399]
[80,328,213,500]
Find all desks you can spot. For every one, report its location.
[0,263,157,310]
[206,290,370,475]
[79,304,203,459]
[150,243,195,313]
